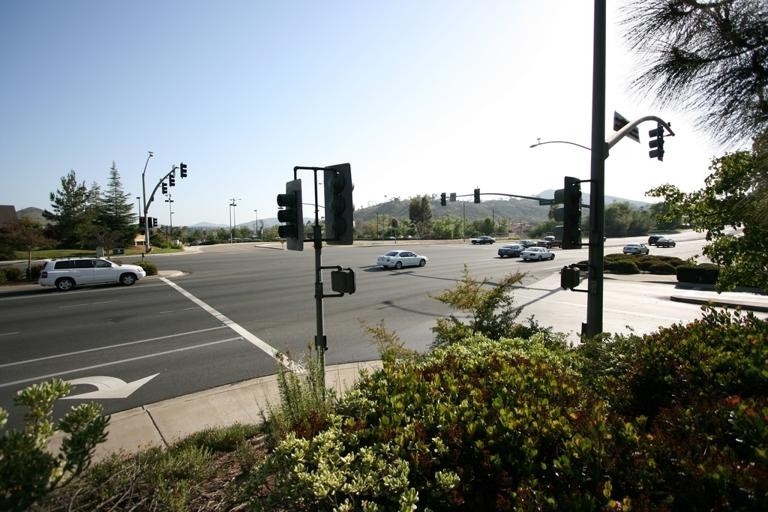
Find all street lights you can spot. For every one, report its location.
[135,192,260,246]
[368,200,382,241]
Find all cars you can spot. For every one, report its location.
[471,234,680,274]
[377,250,429,270]
[384,232,414,240]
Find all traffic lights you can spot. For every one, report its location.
[439,192,446,207]
[169,174,175,187]
[473,188,481,204]
[277,177,304,254]
[559,266,581,290]
[648,127,666,161]
[162,182,167,194]
[553,175,585,249]
[180,161,188,179]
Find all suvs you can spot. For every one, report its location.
[37,254,148,292]
